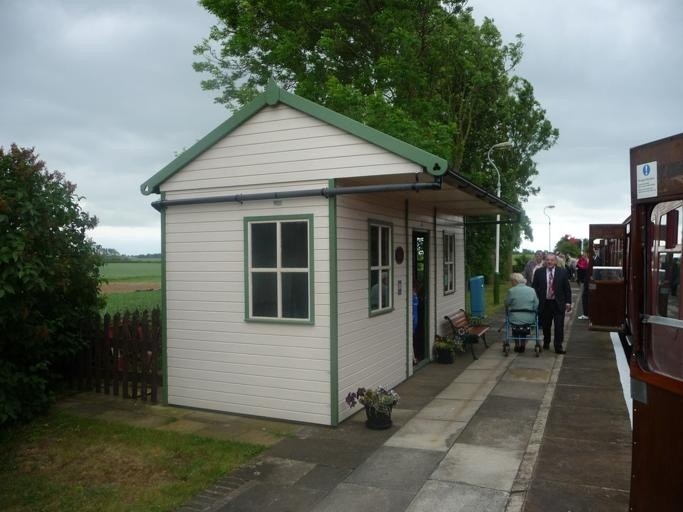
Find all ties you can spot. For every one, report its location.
[547,270,556,299]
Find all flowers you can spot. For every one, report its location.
[346,386,399,418]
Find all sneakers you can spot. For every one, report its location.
[554,346,566,354]
[542,342,549,349]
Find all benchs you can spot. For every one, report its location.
[445,306,490,360]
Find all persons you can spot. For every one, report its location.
[532,254,572,353]
[522,244,600,320]
[412,283,419,365]
[371,271,388,309]
[504,272,540,353]
[651,254,679,317]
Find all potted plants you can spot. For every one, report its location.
[435,339,458,364]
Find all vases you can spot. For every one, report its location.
[365,416,393,430]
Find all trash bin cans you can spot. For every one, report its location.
[470,274,485,316]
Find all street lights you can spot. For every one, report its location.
[543,205,556,251]
[488,141,516,305]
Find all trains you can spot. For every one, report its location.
[584,131,682,510]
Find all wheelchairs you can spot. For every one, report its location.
[500,305,545,358]
